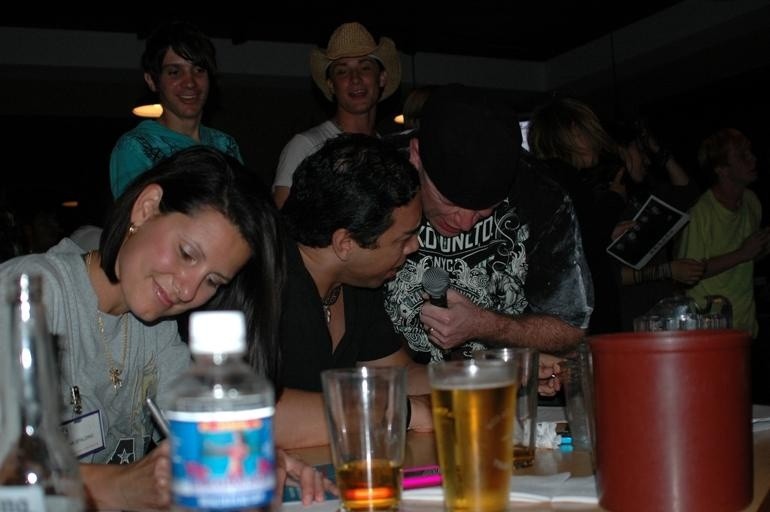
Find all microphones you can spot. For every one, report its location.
[421,267,464,362]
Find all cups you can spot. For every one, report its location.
[318,361,409,512]
[427,361,520,511]
[475,348,541,468]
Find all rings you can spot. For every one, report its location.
[426,327,434,335]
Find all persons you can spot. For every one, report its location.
[273,132,434,450]
[0,145,288,511]
[527,96,769,340]
[108,24,245,202]
[272,22,411,210]
[382,82,594,407]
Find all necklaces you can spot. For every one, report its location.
[320,287,341,326]
[85,249,129,385]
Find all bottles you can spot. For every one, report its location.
[2,267,84,512]
[161,308,280,511]
[559,337,592,456]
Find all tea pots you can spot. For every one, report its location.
[635,291,734,332]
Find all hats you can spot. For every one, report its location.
[308,22,402,104]
[416,82,517,207]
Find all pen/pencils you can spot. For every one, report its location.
[145,396,170,441]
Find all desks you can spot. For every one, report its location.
[399,396,769,511]
